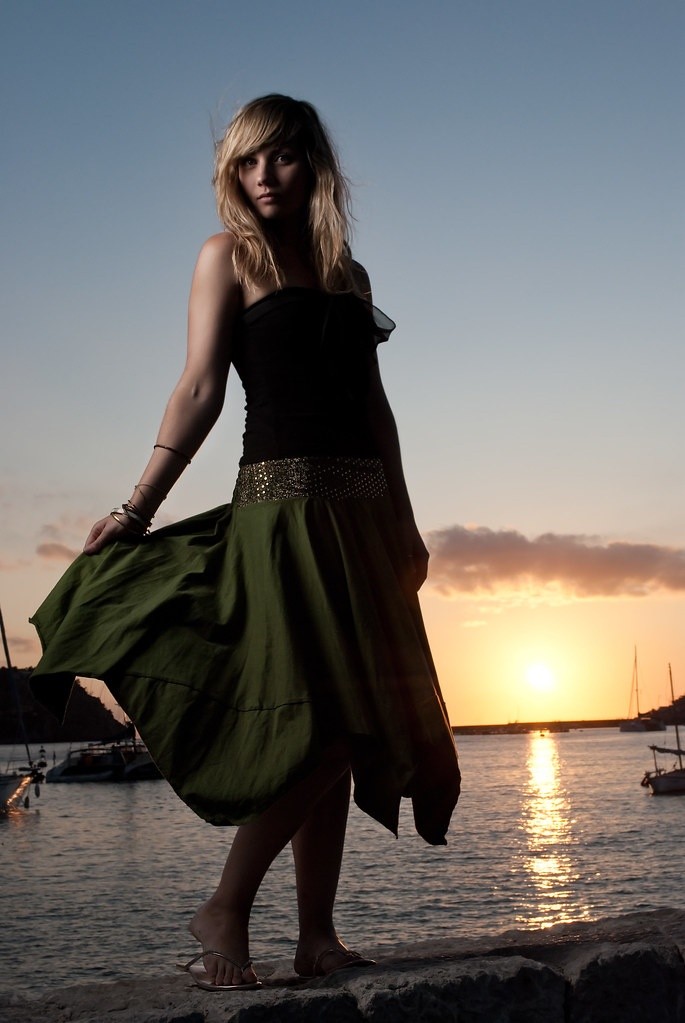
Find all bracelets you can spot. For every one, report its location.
[136,484,167,514]
[153,444,191,464]
[112,501,152,535]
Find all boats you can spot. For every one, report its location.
[549,722,569,732]
[46,676,163,783]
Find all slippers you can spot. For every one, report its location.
[298,947,378,980]
[175,949,264,992]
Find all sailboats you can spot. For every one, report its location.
[0,610,45,819]
[639,663,685,797]
[620,644,668,731]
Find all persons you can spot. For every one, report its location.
[28,94,460,992]
